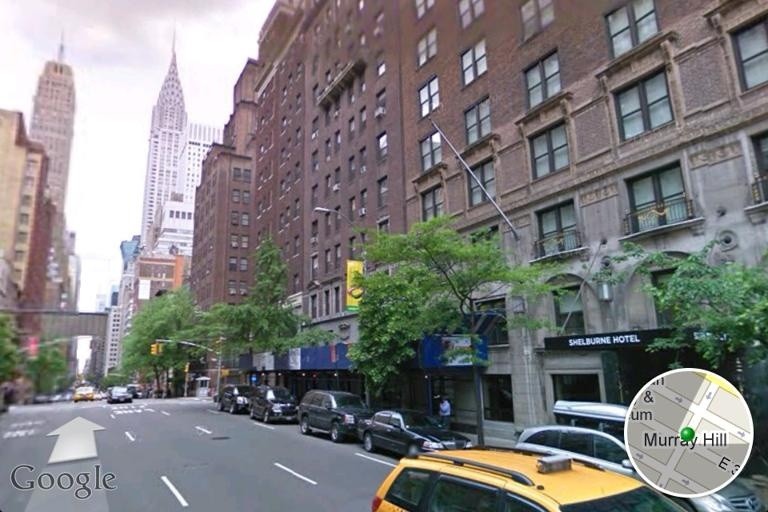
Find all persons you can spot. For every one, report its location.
[438,394,453,430]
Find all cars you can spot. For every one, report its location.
[218,383,297,423]
[34,384,152,404]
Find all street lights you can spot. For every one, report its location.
[312,206,371,387]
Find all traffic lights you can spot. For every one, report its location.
[150,343,158,356]
